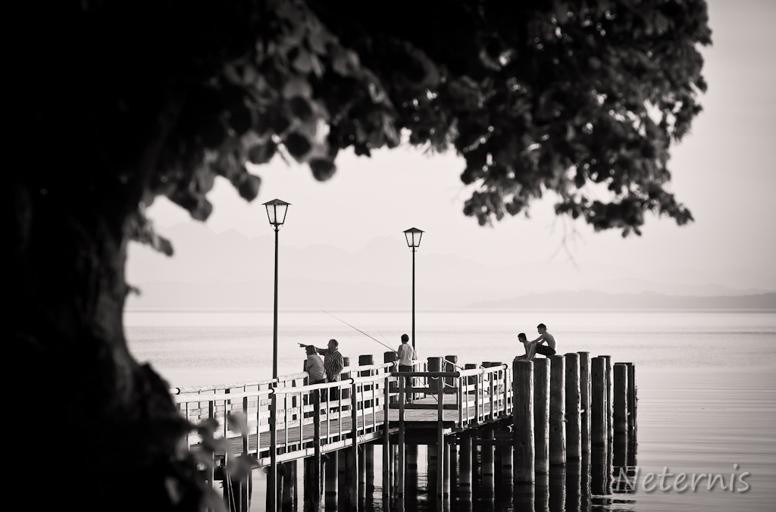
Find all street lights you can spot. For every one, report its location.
[405,227,424,400]
[262,198,291,386]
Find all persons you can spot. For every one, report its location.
[398,334,418,402]
[526,323,556,360]
[305,345,328,416]
[297,339,344,412]
[515,333,530,359]
